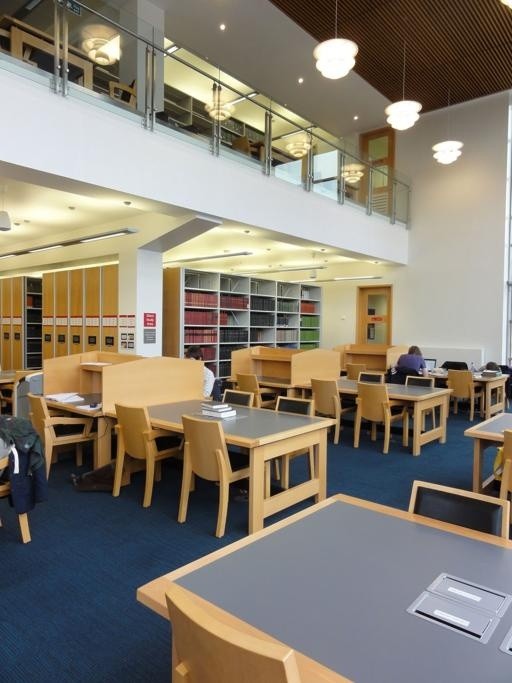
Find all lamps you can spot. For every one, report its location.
[204,86,237,121]
[431,88,464,165]
[384,38,424,130]
[340,162,365,183]
[0,183,12,231]
[311,0,360,80]
[0,226,140,259]
[80,22,122,66]
[286,134,311,157]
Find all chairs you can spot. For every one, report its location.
[357,372,385,385]
[499,430,510,501]
[164,583,302,683]
[447,369,484,422]
[111,402,184,509]
[387,368,424,385]
[221,389,254,456]
[353,383,408,455]
[176,415,271,538]
[0,439,31,544]
[407,479,510,538]
[231,133,252,156]
[102,78,137,109]
[271,396,315,490]
[234,372,280,410]
[395,376,436,432]
[209,380,238,401]
[310,379,357,445]
[344,363,368,380]
[11,371,38,418]
[26,393,98,481]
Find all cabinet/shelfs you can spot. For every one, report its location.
[163,266,322,378]
[25,275,44,369]
[94,48,265,159]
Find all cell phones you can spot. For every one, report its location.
[90,402,96,408]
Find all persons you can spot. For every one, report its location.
[479,362,509,410]
[185,346,216,398]
[396,346,428,377]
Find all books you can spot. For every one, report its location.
[28,281,42,367]
[184,291,321,394]
[201,401,236,418]
[44,392,84,403]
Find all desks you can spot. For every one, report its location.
[293,376,453,457]
[418,369,510,421]
[136,490,511,683]
[0,13,105,90]
[463,412,511,493]
[331,341,409,375]
[43,349,205,488]
[231,346,341,398]
[104,398,337,536]
[251,140,299,163]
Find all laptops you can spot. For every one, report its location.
[419,359,436,372]
